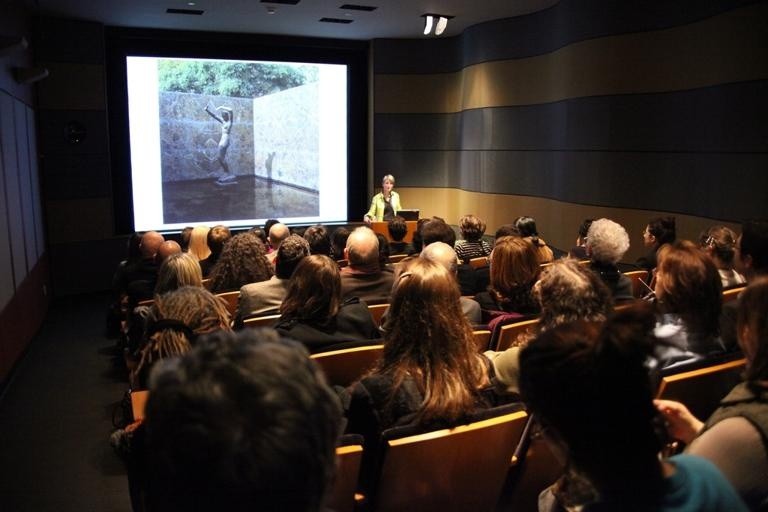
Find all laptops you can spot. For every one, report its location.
[396,209,418,221]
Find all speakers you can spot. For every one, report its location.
[36,109,110,155]
[43,155,112,199]
[0,34,29,59]
[14,66,49,85]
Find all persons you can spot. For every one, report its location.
[362,173,406,223]
[102,214,767,510]
[203,103,238,186]
